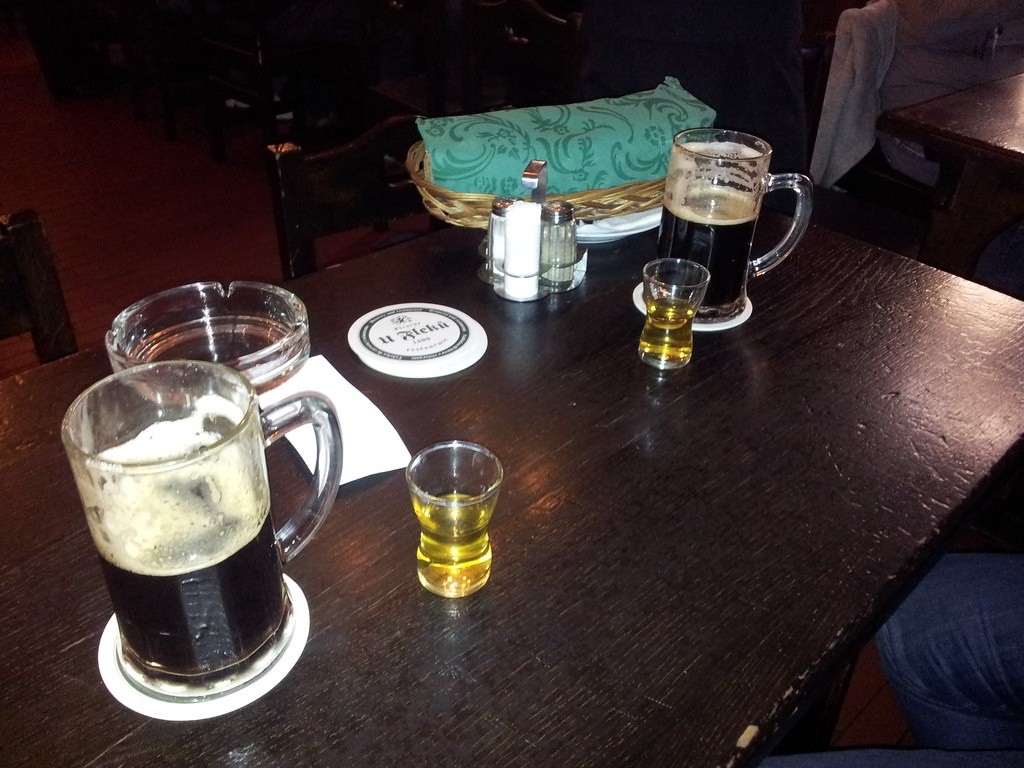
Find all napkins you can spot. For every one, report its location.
[252,355,414,498]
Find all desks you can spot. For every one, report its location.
[0,208,1024,768]
[876,73,1024,270]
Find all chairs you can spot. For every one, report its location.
[267,101,515,281]
[0,211,79,364]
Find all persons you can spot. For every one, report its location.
[745,552,1023,768]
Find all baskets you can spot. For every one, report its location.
[404,139,666,229]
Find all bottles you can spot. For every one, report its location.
[486,194,524,278]
[539,200,576,293]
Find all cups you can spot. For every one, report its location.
[406,440,505,599]
[60,359,343,702]
[655,127,814,324]
[638,258,711,370]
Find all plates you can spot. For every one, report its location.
[576,206,666,244]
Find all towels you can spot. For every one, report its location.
[810,0,900,191]
